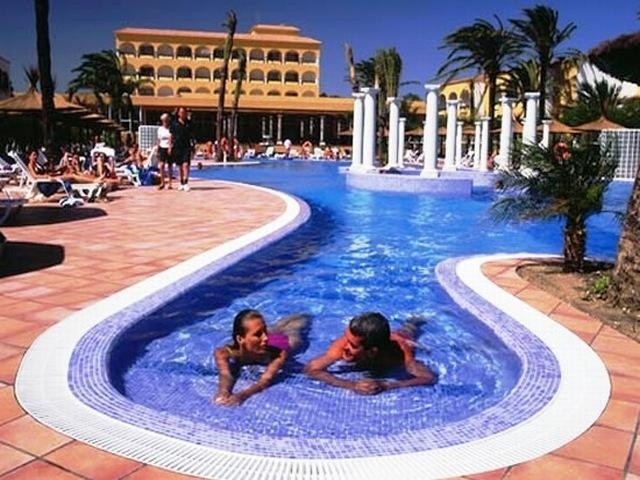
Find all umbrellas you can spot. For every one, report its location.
[338,115,629,154]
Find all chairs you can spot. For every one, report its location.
[244,145,351,160]
[0,142,160,244]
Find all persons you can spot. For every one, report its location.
[488,137,572,171]
[307,315,439,395]
[403,145,419,163]
[1,131,157,195]
[202,135,351,162]
[170,107,196,191]
[209,307,316,406]
[157,112,174,192]
